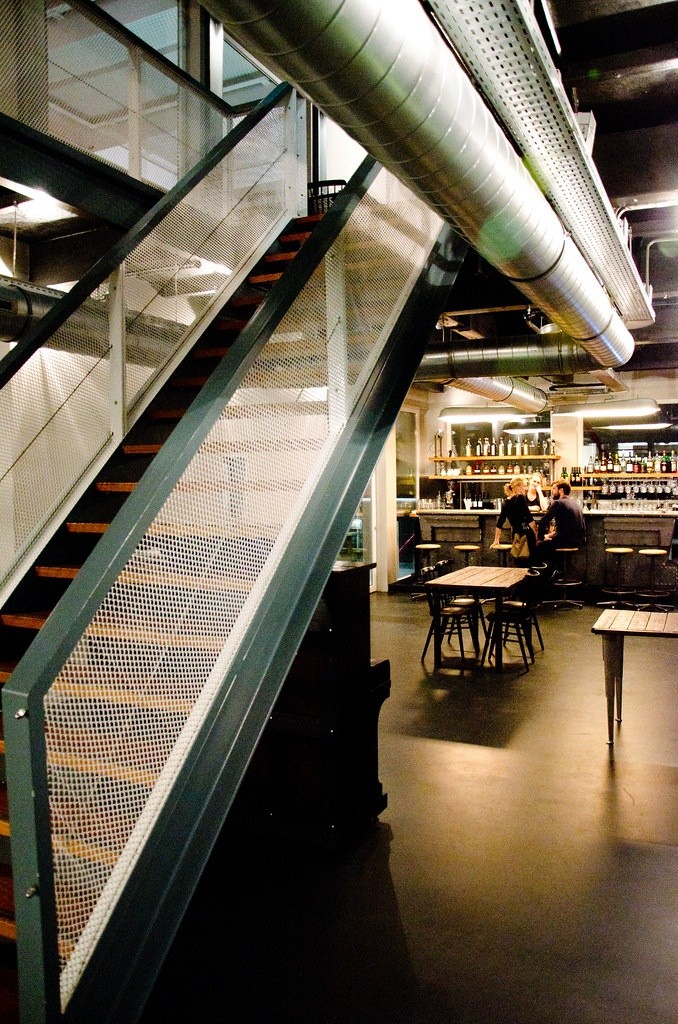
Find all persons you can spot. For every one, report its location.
[487,476,542,569]
[536,479,586,599]
[522,473,549,513]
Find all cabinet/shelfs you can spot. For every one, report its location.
[427,434,678,510]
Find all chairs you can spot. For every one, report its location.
[420,559,548,674]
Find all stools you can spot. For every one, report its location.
[541,547,678,613]
[411,543,513,601]
[343,532,358,561]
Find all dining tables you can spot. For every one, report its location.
[425,565,530,675]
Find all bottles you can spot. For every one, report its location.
[563,450,677,515]
[420,490,503,510]
[463,435,549,474]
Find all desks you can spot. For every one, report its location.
[355,507,419,563]
[591,609,678,745]
[417,508,678,600]
